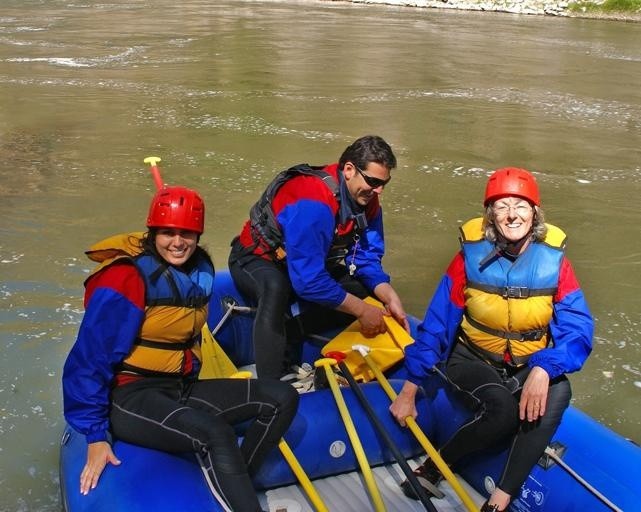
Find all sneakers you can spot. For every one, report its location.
[481,499,510,512]
[401,457,445,500]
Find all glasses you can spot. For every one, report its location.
[353,162,391,187]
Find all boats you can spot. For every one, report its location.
[59,271,641,512]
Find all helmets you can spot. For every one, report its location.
[145,186,204,233]
[484,167,541,207]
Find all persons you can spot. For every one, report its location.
[390,168,594,512]
[62,185,300,512]
[228,136,411,384]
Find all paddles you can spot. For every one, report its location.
[144,157,239,379]
